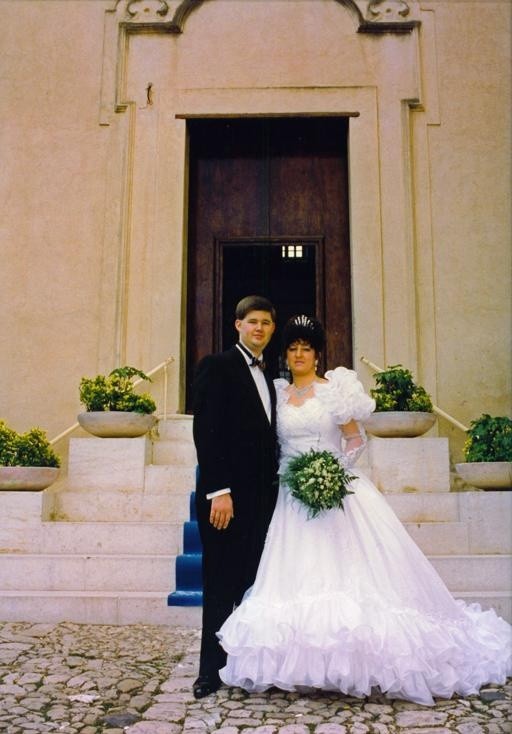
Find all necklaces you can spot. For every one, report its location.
[289,380,317,398]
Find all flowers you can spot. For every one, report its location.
[275,441,359,524]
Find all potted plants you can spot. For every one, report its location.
[361,366,512,490]
[0,363,166,493]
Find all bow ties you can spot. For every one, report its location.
[249,357,266,371]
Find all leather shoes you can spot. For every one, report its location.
[194,679,222,699]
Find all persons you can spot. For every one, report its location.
[190,295,279,699]
[213,313,512,706]
